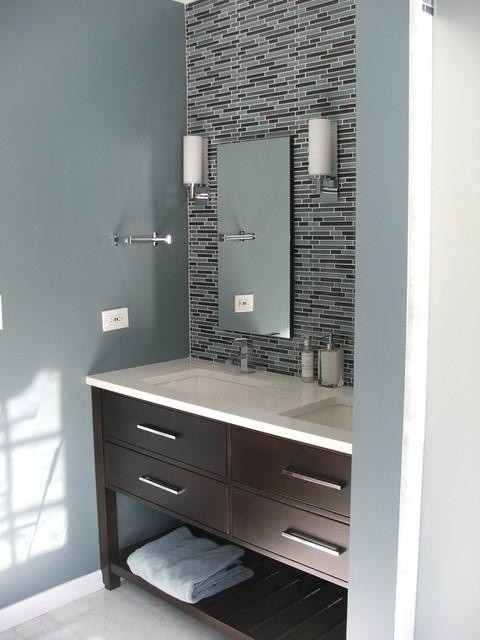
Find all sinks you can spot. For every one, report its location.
[143,369,273,409]
[277,394,355,439]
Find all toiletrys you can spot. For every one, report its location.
[318,333,344,387]
[299,339,316,384]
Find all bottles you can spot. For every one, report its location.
[301,340,314,383]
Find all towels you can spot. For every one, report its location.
[128,526,254,604]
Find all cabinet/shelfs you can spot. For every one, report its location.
[90,385,352,640]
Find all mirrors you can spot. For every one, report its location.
[218,136,293,339]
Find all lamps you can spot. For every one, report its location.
[183,135,207,201]
[307,119,338,194]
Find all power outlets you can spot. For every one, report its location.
[101,307,129,332]
[235,295,254,312]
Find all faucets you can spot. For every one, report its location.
[235,338,256,373]
[261,331,281,335]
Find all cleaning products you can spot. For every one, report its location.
[302,339,315,380]
